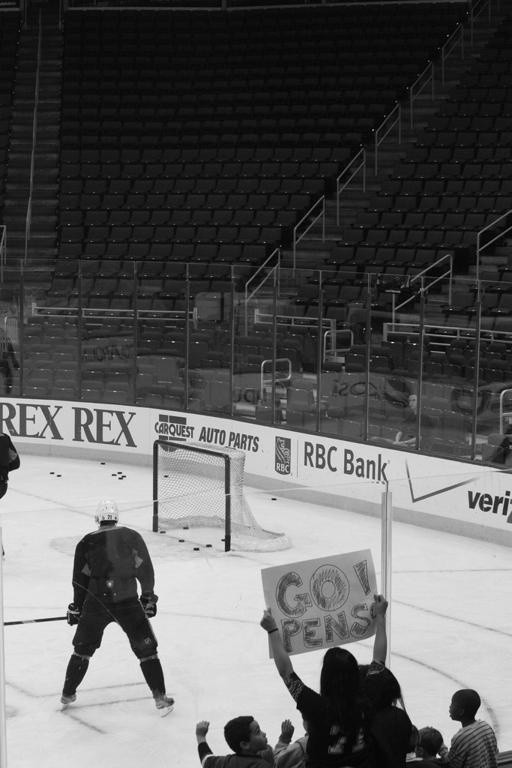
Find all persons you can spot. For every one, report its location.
[62,504,174,709]
[196,597,498,768]
[0,431,20,555]
[492,423,512,464]
[0,326,22,394]
[393,395,424,450]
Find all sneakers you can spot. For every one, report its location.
[61,694,76,703]
[155,696,174,709]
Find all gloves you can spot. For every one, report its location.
[67,603,81,625]
[140,594,158,616]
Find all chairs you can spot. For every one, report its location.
[1,1,512,474]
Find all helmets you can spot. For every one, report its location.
[96,500,118,523]
